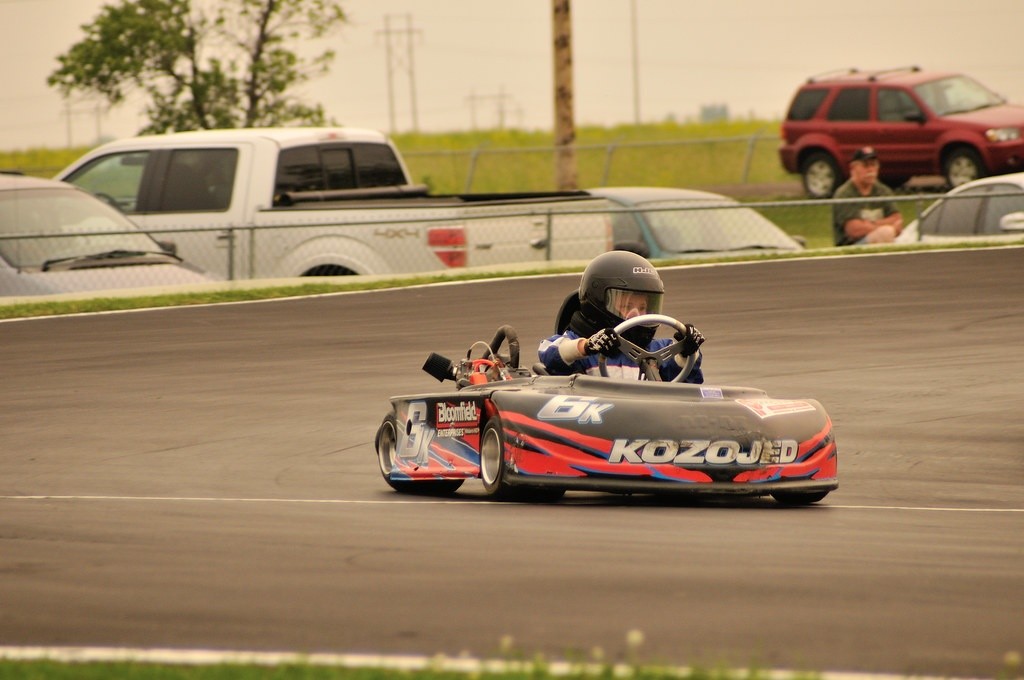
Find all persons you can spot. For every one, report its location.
[830,145,904,246]
[539,250,706,389]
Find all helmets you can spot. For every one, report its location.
[579,250,664,350]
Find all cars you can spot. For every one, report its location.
[1,172,228,310]
[892,173,1024,248]
[588,181,812,263]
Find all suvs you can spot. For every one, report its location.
[774,66,1024,190]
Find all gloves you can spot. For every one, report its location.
[673,324,705,358]
[584,329,623,360]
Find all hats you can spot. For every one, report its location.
[848,147,879,164]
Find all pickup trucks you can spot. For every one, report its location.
[52,122,613,290]
